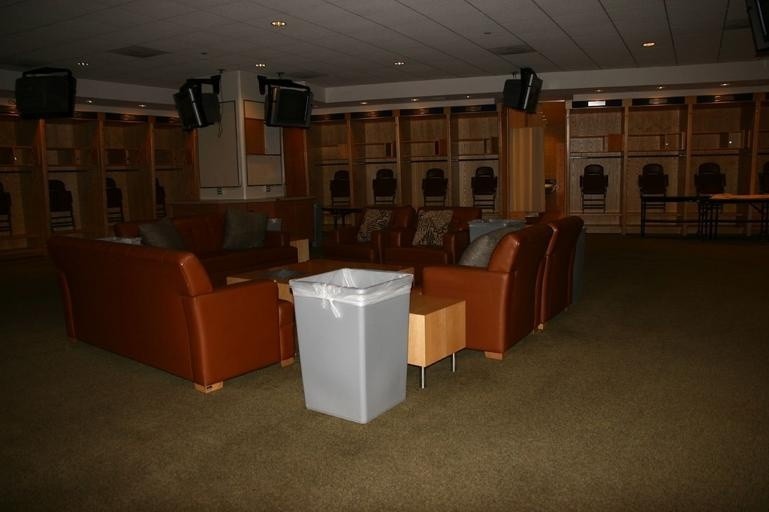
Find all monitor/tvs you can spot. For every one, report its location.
[258,74,314,129]
[503,67,544,115]
[172,78,220,131]
[15,68,76,119]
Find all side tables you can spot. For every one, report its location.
[407,296,468,391]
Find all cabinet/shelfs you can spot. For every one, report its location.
[568,95,768,238]
[0,105,197,260]
[303,105,502,224]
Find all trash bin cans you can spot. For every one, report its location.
[469,218,522,245]
[289,267,414,424]
